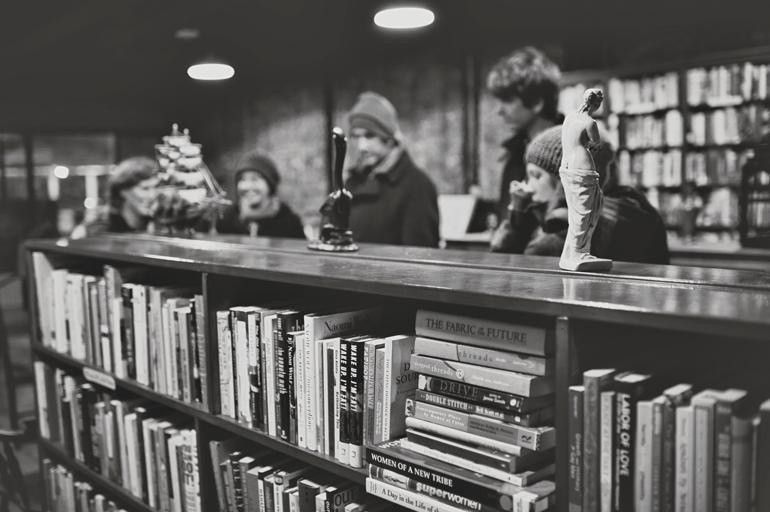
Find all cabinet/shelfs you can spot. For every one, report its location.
[19,42,768,512]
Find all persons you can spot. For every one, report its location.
[484,43,565,233]
[339,91,442,248]
[485,126,672,264]
[81,153,159,237]
[213,148,308,241]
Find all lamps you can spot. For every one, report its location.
[368,4,441,39]
[186,4,238,84]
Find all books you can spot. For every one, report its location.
[0,250,770,512]
[553,58,770,250]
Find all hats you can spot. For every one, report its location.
[234,149,280,194]
[525,126,614,191]
[348,91,398,139]
